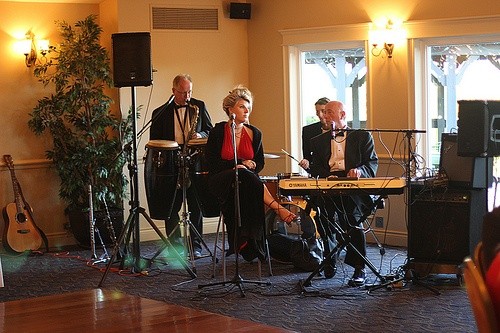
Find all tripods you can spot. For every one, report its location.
[98,87,271,298]
[334,129,442,296]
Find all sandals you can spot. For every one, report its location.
[268,199,298,224]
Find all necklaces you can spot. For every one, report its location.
[235,126,245,138]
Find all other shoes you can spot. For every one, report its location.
[168,246,184,257]
[193,251,201,257]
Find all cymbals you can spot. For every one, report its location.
[264,153,280,159]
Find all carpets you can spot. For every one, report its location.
[0,231,478,333]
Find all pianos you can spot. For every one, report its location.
[279,175,408,294]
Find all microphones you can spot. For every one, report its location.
[330,121,336,139]
[229,113,237,120]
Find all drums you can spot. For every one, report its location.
[259,175,281,212]
[188,138,220,217]
[277,173,302,198]
[144,140,181,219]
[266,196,317,264]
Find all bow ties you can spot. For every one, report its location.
[330,132,344,139]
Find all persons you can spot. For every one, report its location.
[301,97,378,286]
[151,75,213,258]
[204,85,294,253]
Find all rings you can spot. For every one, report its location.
[250,163,252,165]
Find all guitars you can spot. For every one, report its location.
[3,155,43,255]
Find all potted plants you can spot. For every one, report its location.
[27,12,142,249]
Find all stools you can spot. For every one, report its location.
[212,208,272,286]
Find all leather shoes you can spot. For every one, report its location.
[349,270,368,285]
[324,255,337,278]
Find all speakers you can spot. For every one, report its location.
[111,32,153,88]
[229,2,251,20]
[408,100,500,263]
